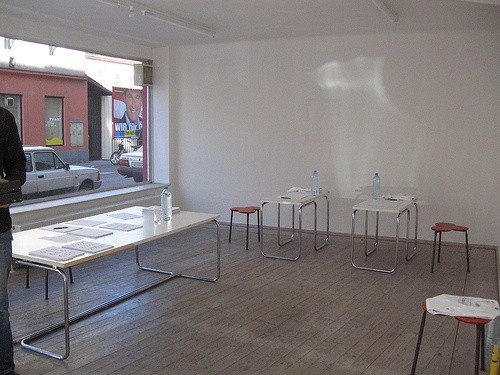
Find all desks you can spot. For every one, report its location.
[260,192,329,261]
[11,205,220,361]
[351,199,418,273]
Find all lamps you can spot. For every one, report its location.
[142,9,216,37]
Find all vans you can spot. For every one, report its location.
[116,146,143,178]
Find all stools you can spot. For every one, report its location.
[409,294,500,375]
[229,207,261,250]
[431,223,470,273]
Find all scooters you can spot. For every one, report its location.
[109,141,141,164]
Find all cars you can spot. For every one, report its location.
[20,146,102,199]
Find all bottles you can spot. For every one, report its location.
[371,173,381,200]
[310,170,319,195]
[160,186,172,221]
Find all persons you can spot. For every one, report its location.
[117,89,143,130]
[0,105,27,375]
